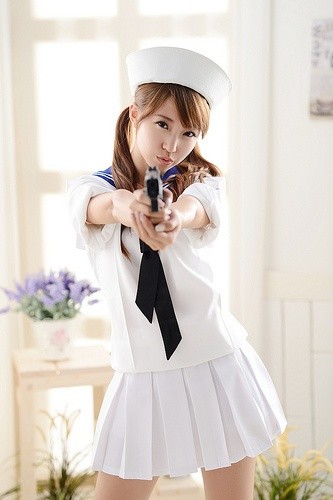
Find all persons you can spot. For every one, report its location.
[69,46,287,500]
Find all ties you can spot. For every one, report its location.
[135,237,181,359]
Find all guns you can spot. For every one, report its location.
[145,166,164,212]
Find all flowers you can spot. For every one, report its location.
[0,269,101,321]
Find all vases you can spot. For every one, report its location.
[34,319,75,361]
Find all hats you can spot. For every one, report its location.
[125,48,232,110]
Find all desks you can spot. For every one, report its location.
[11,338,116,500]
